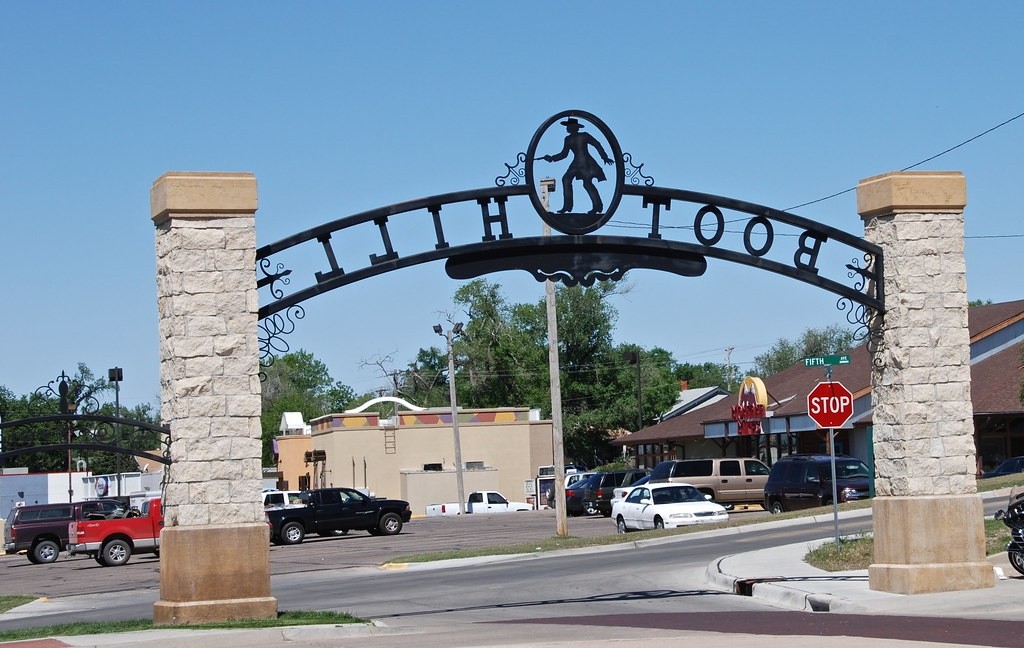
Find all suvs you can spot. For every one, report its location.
[545,469,584,510]
[764,452,871,515]
[584,469,649,518]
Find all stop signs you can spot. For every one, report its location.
[807,380,853,429]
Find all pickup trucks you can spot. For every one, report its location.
[66,497,164,567]
[3,495,141,565]
[264,487,413,546]
[424,490,534,517]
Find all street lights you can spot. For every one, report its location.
[108,366,124,498]
[432,322,468,515]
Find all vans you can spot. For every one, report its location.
[648,458,772,506]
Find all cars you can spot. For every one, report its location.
[981,456,1024,478]
[565,472,606,516]
[611,481,730,533]
[610,475,653,507]
[262,488,312,510]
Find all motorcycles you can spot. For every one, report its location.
[994,484,1024,576]
